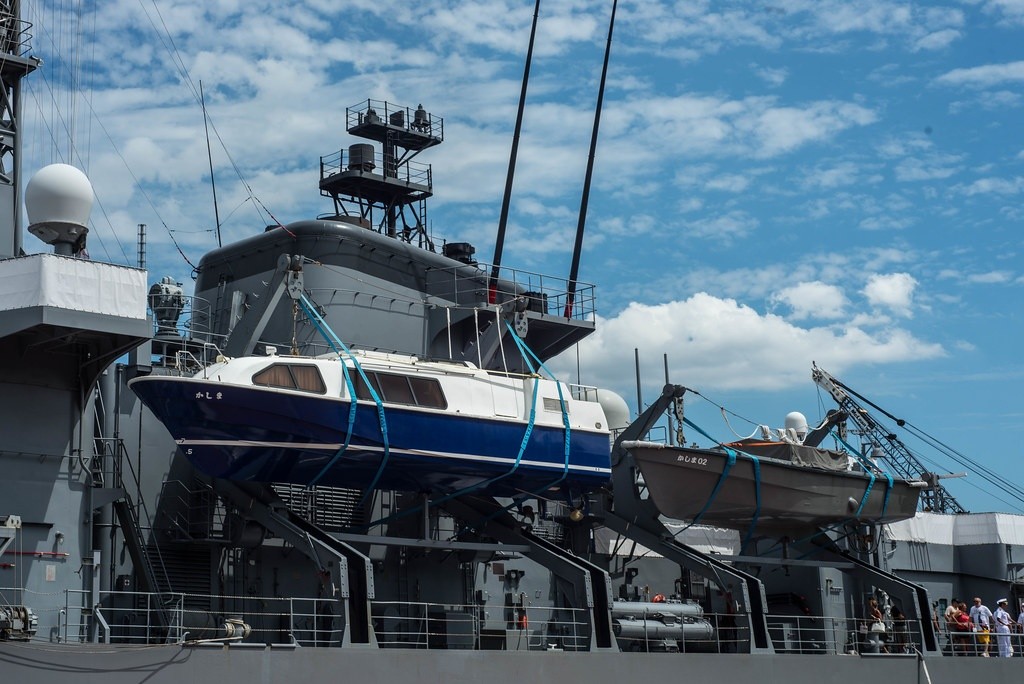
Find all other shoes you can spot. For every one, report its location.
[980,651,990,657]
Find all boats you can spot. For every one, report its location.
[622,434,930,527]
[125,348,631,494]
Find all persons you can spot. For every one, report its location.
[865,595,890,653]
[890,605,913,652]
[994,598,1024,658]
[968,597,996,657]
[931,602,942,635]
[945,598,973,656]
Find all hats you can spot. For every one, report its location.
[997,598,1008,605]
[933,601,939,608]
[952,598,961,603]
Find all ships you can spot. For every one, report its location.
[0,0,1024,684]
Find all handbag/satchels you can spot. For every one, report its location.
[870,618,885,633]
